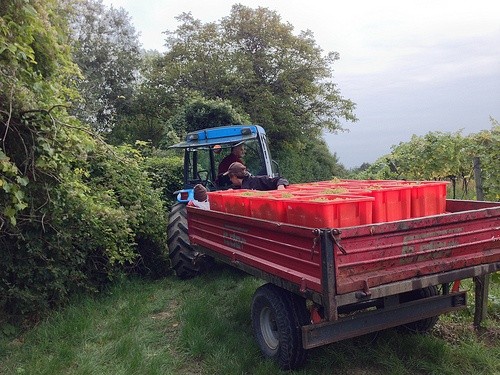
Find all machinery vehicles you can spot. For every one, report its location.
[166,125,500,368]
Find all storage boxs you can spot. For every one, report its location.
[206,179,451,229]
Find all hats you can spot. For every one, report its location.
[223,162,247,176]
[193,183,207,202]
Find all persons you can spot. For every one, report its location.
[223,162,289,190]
[216,143,245,189]
